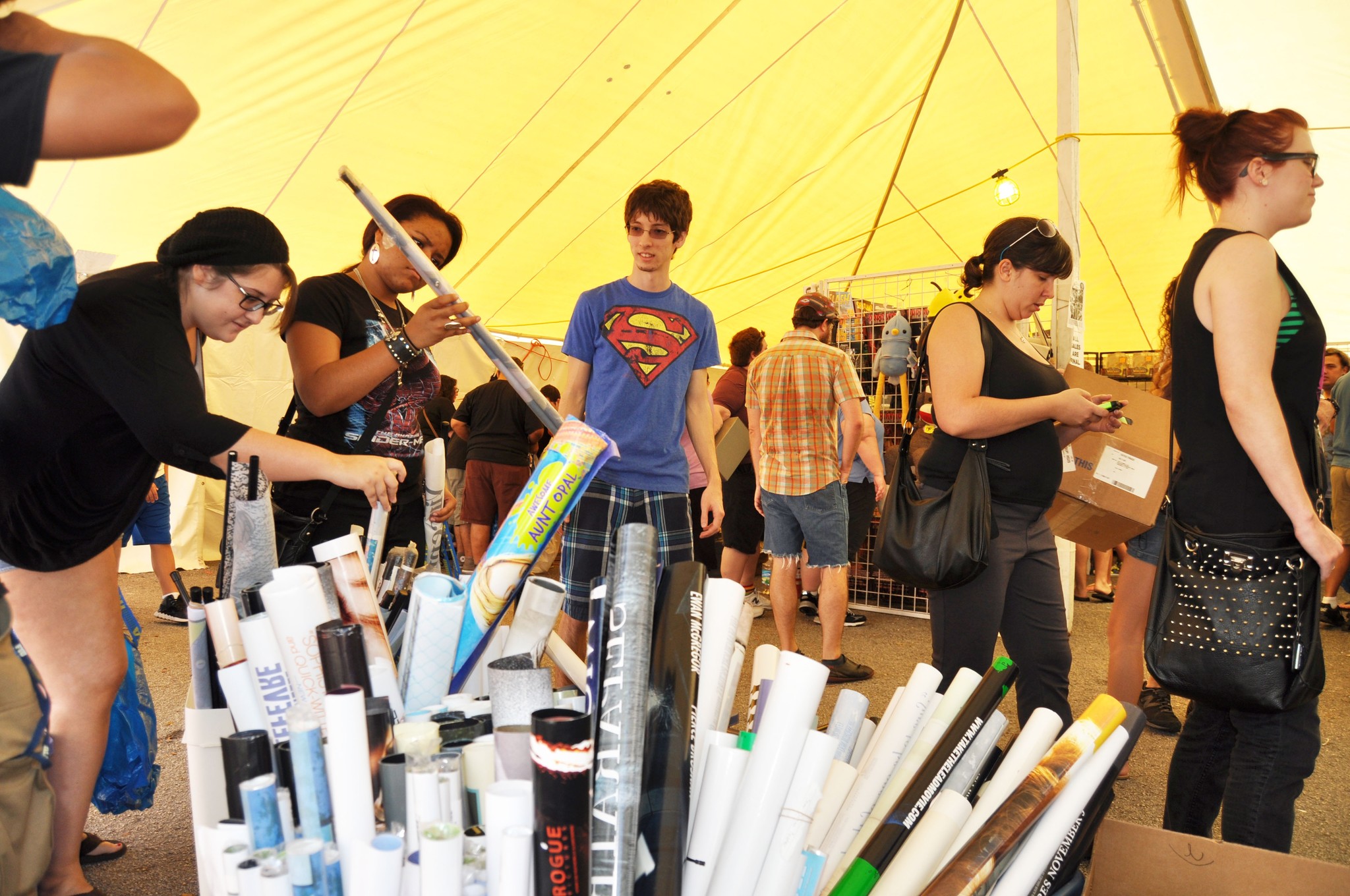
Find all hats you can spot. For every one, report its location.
[794,292,838,320]
[157,207,288,265]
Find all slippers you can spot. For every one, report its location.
[37,880,103,896]
[80,831,125,864]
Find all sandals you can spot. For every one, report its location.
[1337,601,1350,611]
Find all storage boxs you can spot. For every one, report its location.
[1053,365,1178,552]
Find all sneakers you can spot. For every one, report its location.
[1138,681,1181,732]
[154,593,188,622]
[456,552,465,568]
[462,564,476,574]
[745,588,773,610]
[799,592,819,616]
[744,600,764,618]
[1319,602,1345,627]
[822,654,874,683]
[814,608,866,626]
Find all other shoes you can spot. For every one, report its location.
[1074,549,1122,602]
[1117,762,1129,779]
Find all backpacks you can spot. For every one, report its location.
[9,585,160,818]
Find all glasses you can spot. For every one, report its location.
[998,219,1056,261]
[219,264,283,316]
[625,224,676,240]
[1240,152,1319,179]
[757,331,765,347]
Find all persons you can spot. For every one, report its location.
[1150,110,1344,854]
[0,13,1350,896]
[2,207,406,896]
[914,219,1130,750]
[745,293,874,684]
[557,179,723,706]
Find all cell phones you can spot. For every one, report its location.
[1097,400,1124,412]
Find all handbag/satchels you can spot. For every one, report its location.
[1143,515,1326,712]
[873,303,990,587]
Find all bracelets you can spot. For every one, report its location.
[382,328,424,389]
